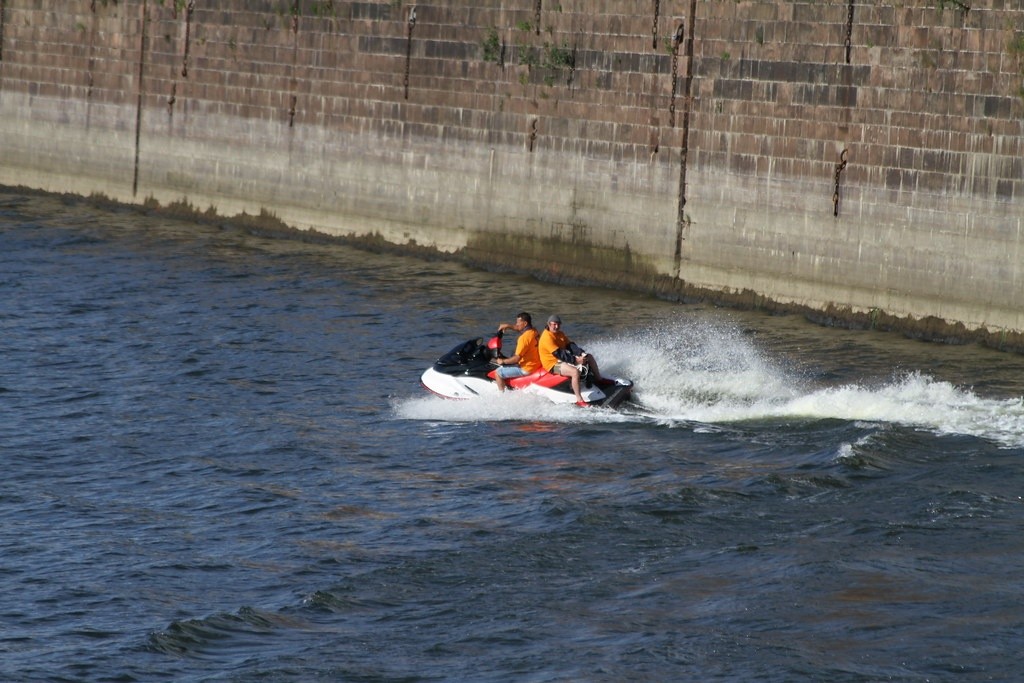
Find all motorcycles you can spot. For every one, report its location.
[420,326,633,408]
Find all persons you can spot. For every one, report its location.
[538,314,590,408]
[494,312,542,390]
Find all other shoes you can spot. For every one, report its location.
[576,400,590,408]
[599,377,614,385]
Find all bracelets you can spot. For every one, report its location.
[502,358,505,364]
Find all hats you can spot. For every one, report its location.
[548,315,562,323]
